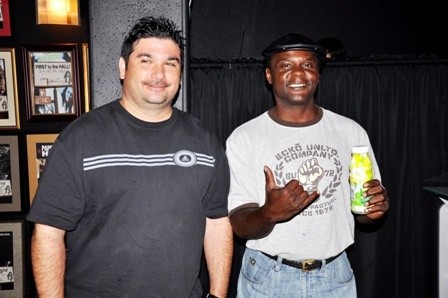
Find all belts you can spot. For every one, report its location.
[261,251,345,273]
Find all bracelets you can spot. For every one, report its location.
[206,293,218,298]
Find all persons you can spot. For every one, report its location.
[26,14,232,298]
[225,33,389,298]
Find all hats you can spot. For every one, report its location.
[262,33,323,58]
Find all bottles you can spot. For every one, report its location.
[348,146,375,214]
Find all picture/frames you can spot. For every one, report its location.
[0,0,91,298]
